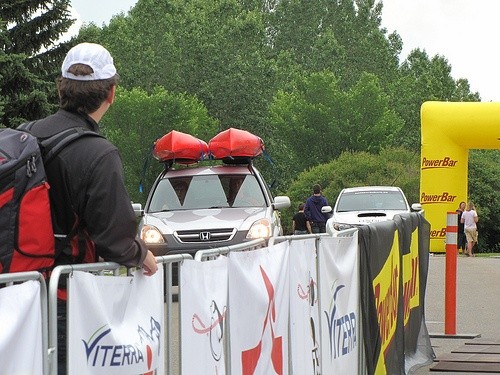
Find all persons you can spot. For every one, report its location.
[16,43,168,375]
[456,201,467,256]
[292,204,313,235]
[460,201,478,257]
[304,185,331,233]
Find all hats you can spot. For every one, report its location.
[61,42,116,81]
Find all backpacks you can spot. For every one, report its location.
[0,121,107,288]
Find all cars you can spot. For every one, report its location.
[321,186,423,236]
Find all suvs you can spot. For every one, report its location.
[132,159,291,264]
